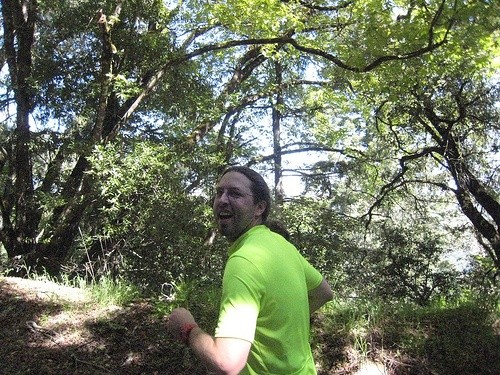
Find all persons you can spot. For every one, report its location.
[168,165,335,375]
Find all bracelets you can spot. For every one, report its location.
[179,324,197,346]
